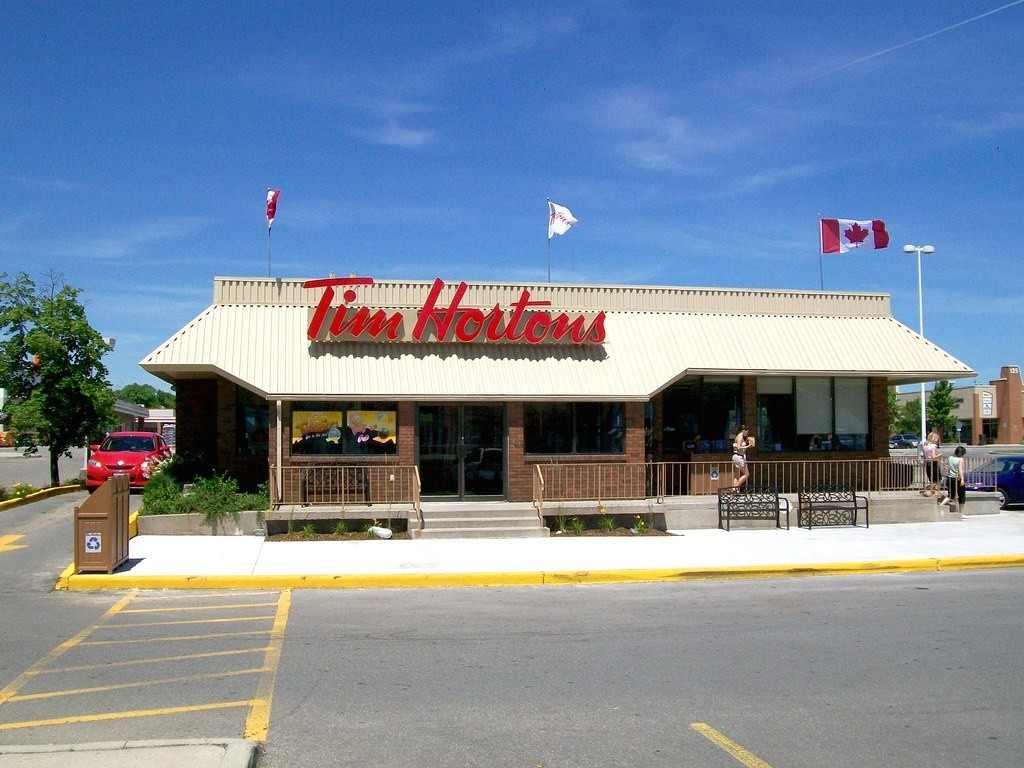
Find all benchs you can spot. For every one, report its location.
[717,486,790,532]
[301,462,372,508]
[797,484,869,530]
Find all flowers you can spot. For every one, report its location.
[632,514,648,534]
[599,509,616,531]
[371,516,383,528]
[572,518,585,536]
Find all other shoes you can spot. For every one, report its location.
[961,514,969,519]
[733,487,740,493]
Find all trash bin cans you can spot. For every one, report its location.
[72,474,130,575]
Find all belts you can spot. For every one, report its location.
[734,452,744,457]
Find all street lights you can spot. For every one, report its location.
[902,244,937,442]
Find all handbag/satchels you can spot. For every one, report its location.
[918,448,925,465]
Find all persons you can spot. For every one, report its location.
[731,425,753,494]
[809,435,849,452]
[937,446,969,518]
[922,432,943,497]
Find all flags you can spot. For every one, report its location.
[547,201,579,240]
[820,217,890,254]
[266,190,280,229]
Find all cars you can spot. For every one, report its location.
[86,431,171,494]
[888,434,923,449]
[0,428,50,447]
[939,453,1024,510]
[466,446,504,495]
